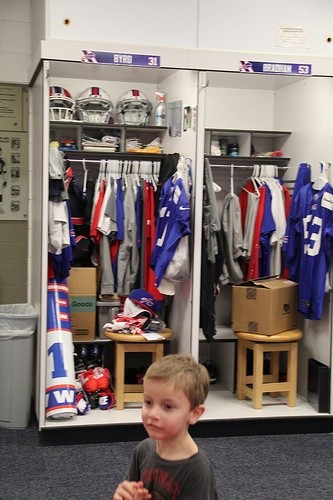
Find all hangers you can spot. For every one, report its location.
[227,160,329,197]
[80,158,193,192]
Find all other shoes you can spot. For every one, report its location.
[200,359,217,385]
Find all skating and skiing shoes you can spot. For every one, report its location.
[78,366,111,409]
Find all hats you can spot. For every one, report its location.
[128,289,157,316]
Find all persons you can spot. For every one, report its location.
[113,354,219,500]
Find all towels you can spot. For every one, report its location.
[48,199,78,285]
[48,146,71,255]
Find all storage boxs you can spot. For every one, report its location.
[232,274,298,337]
[67,267,98,342]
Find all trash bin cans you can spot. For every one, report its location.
[0,302,38,431]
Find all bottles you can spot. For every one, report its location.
[211,141,221,156]
[218,139,228,156]
[228,143,239,156]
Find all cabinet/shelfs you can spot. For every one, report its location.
[204,128,291,162]
[49,119,167,156]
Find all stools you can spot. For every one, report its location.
[235,330,302,411]
[104,328,171,411]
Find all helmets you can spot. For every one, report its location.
[115,88,153,127]
[75,86,113,124]
[49,86,75,120]
